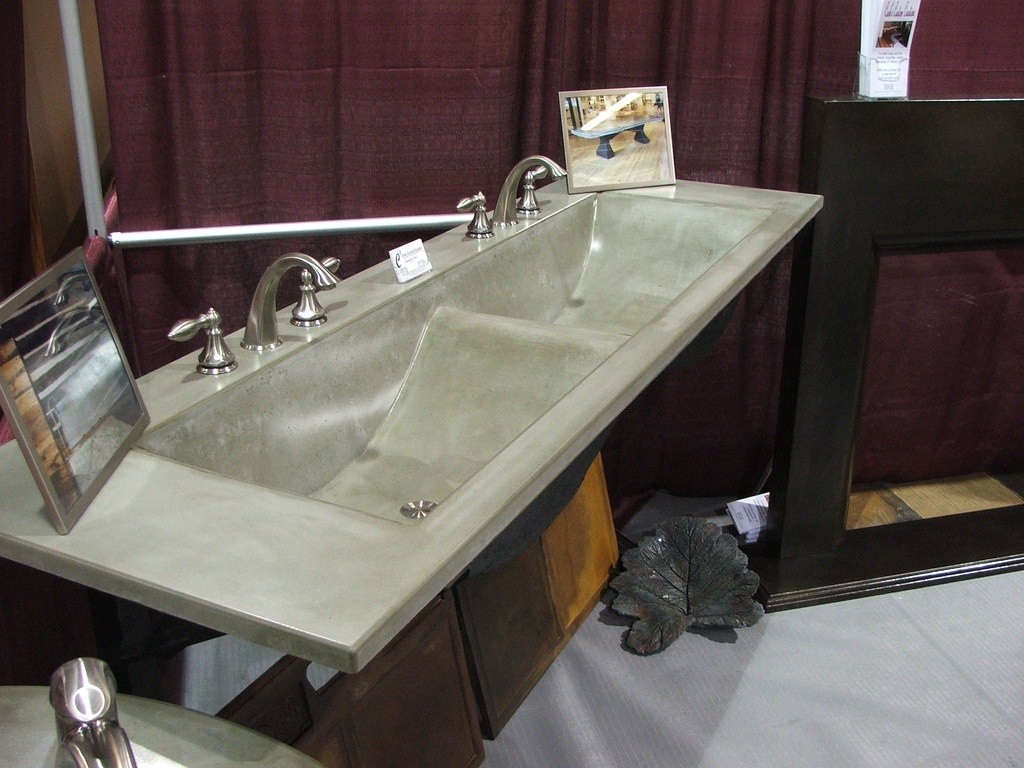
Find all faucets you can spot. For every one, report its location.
[489,154,569,227]
[47,656,140,768]
[239,252,341,352]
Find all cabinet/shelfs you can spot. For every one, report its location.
[454,450,620,742]
[212,584,486,768]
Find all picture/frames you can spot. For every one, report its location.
[558,85,676,194]
[0,246,150,535]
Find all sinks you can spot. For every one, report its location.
[135,191,776,528]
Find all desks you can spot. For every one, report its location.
[571,115,662,159]
